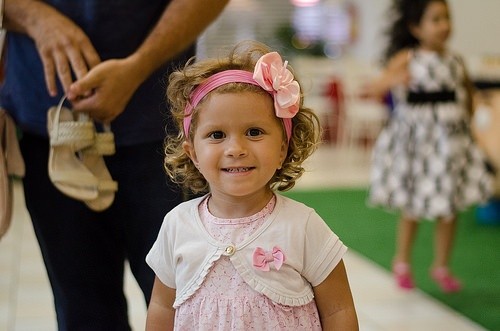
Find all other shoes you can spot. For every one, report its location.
[394,262,413,289]
[433,266,459,292]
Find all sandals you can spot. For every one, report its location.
[70,108,118,212]
[47,96,99,200]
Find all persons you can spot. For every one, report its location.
[142,40,362,331]
[368,0,498,292]
[0,0,234,331]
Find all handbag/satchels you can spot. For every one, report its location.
[0,107,25,239]
